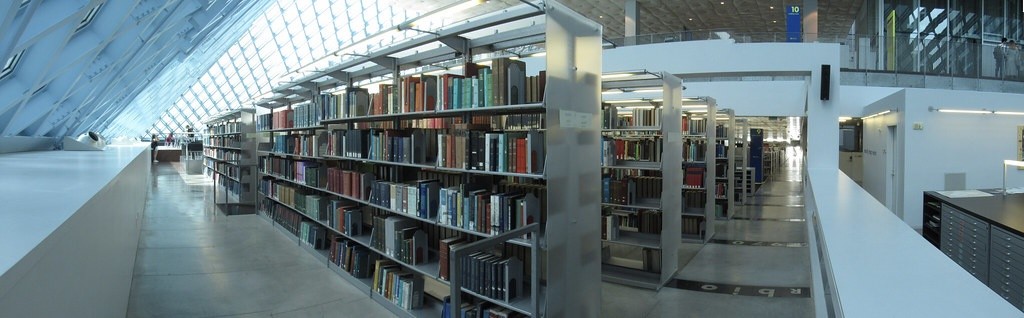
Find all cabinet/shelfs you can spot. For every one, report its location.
[201,0,748,318]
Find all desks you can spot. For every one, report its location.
[923,187,1024,314]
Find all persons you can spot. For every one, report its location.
[167,132,175,146]
[994,37,1021,79]
[186,125,194,137]
[150,134,159,162]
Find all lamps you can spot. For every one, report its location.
[928,106,1024,115]
[1003,159,1024,195]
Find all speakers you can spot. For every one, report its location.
[820,65,831,100]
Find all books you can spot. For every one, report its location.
[201,58,546,318]
[599,102,781,274]
